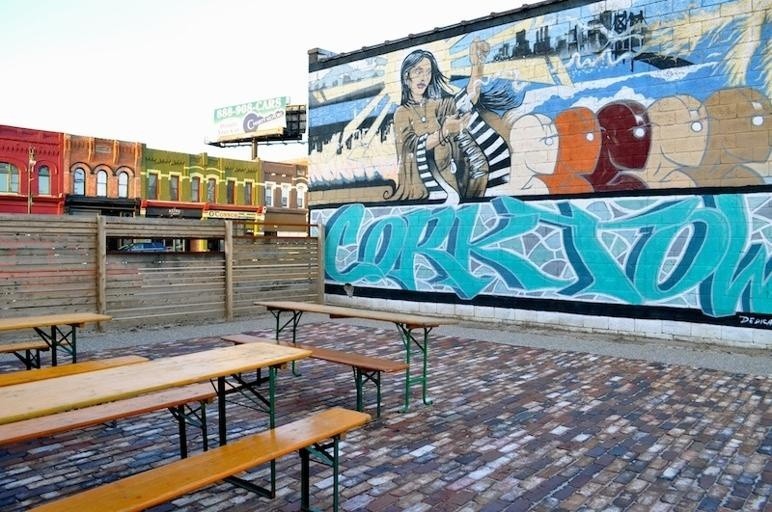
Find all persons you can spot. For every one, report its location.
[582,100,651,191]
[487,113,560,195]
[682,88,772,187]
[535,108,602,194]
[382,36,510,201]
[621,95,708,190]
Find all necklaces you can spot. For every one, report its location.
[412,99,428,124]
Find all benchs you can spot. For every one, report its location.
[0,355,150,386]
[27,406,371,510]
[221,333,410,417]
[1,381,245,452]
[0,338,72,369]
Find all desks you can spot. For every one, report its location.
[253,299,460,413]
[0,312,113,365]
[1,341,312,498]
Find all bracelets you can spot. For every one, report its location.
[437,129,447,146]
[440,126,450,142]
